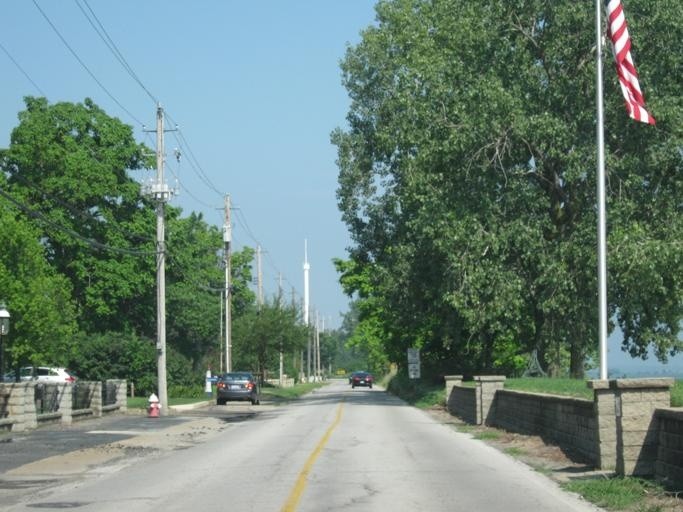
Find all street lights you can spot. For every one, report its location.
[0,306,12,339]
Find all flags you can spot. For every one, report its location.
[603,0,657,126]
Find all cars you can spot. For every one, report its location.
[216,372,262,405]
[348,369,376,389]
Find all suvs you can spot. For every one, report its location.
[2,365,79,385]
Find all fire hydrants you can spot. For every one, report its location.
[147,393,162,417]
